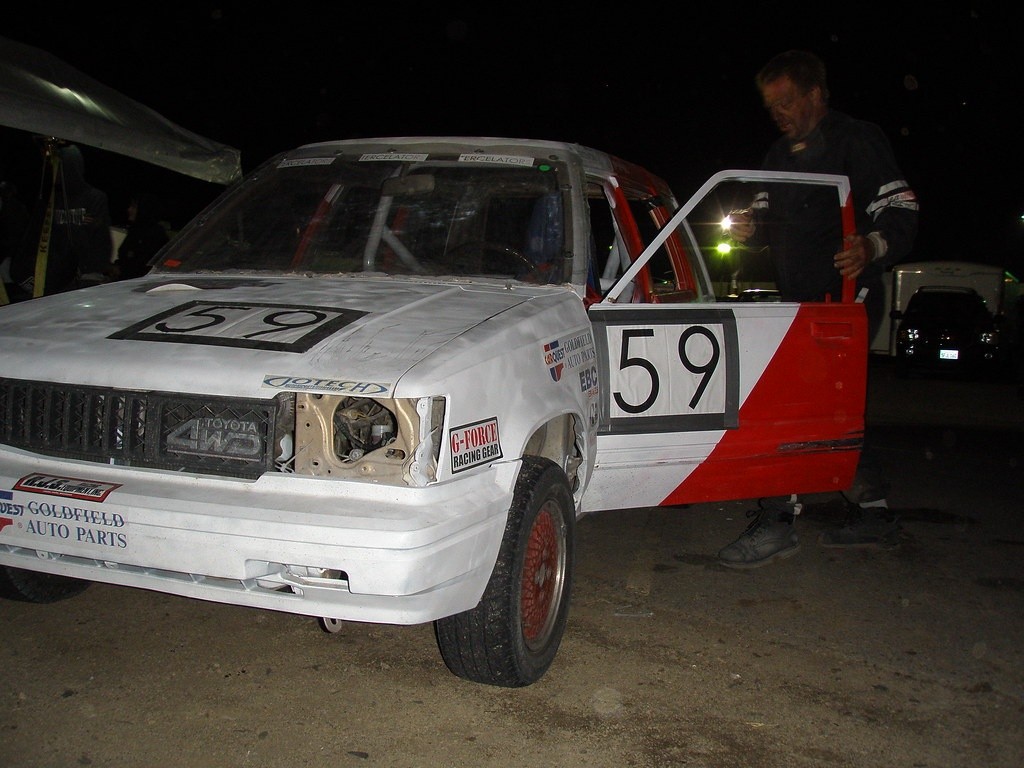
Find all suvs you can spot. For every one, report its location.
[888,286,1008,383]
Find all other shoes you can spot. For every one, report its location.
[817,511,896,548]
[717,508,802,570]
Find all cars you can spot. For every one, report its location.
[0,132,868,688]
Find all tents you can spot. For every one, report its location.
[0,35,244,256]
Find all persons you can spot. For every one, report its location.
[12,145,112,294]
[715,51,919,569]
[0,181,38,304]
[113,188,169,276]
